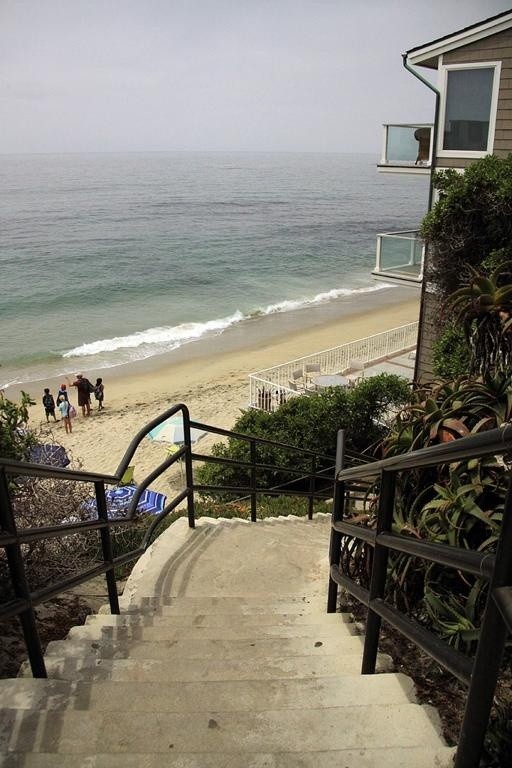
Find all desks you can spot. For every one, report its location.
[311,375,350,391]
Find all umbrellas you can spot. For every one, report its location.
[15,442,69,483]
[148,412,209,486]
[81,485,167,519]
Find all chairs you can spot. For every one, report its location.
[346,360,365,385]
[286,361,323,392]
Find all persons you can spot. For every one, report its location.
[59,395,72,434]
[43,389,60,423]
[57,384,67,420]
[65,372,104,417]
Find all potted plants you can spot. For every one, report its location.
[255,381,275,410]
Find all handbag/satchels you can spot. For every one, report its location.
[89,385,94,393]
[67,405,76,418]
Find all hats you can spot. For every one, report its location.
[75,373,82,376]
[61,384,66,389]
